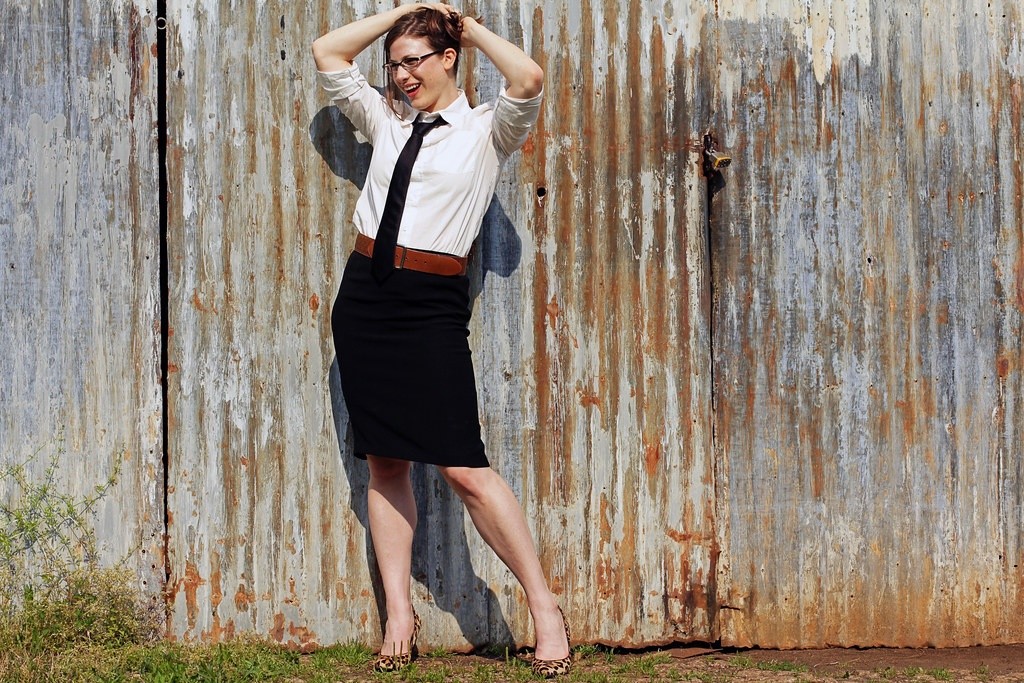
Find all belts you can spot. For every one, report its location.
[354,232,468,276]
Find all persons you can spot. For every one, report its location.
[312,3,573,677]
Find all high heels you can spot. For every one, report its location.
[531,604,572,679]
[375,604,421,671]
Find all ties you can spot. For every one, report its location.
[372,113,449,284]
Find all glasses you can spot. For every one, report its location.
[381,48,444,74]
